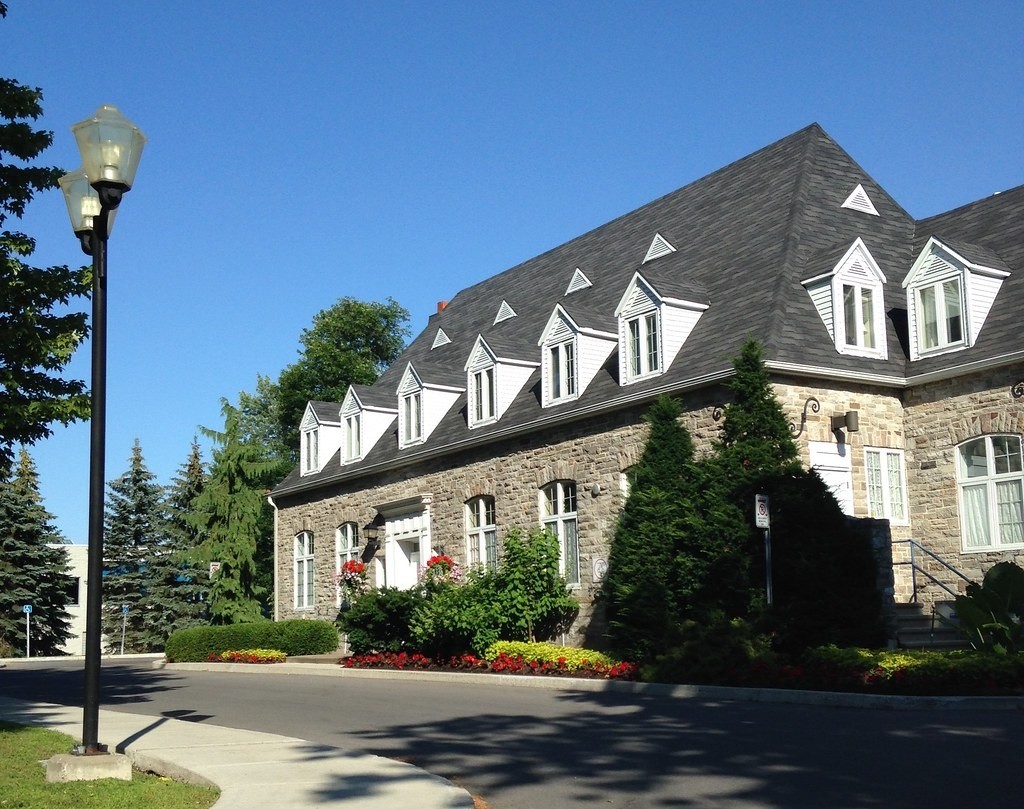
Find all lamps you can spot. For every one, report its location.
[363,523,381,549]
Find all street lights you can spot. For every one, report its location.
[56,101,149,760]
